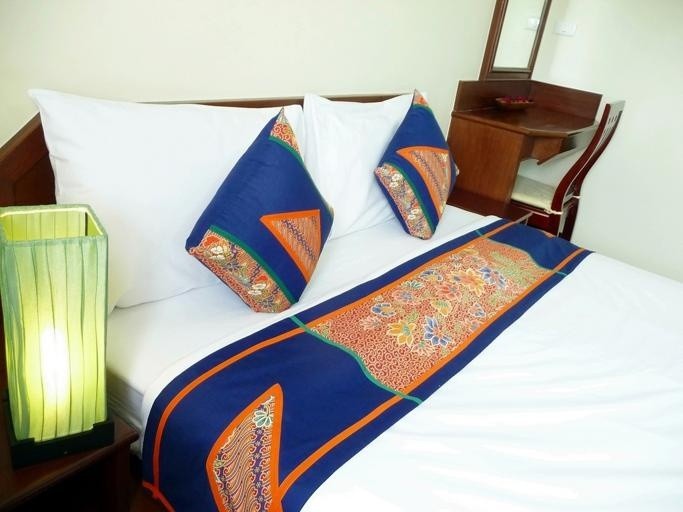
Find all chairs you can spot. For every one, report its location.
[507,98,625,243]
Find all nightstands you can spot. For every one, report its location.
[0,398,140,511]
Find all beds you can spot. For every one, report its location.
[0,95,683,510]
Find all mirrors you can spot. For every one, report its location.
[479,0,552,83]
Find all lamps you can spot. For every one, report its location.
[0,199,114,466]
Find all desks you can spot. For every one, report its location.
[443,79,602,219]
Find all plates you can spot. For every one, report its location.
[494,96,535,111]
